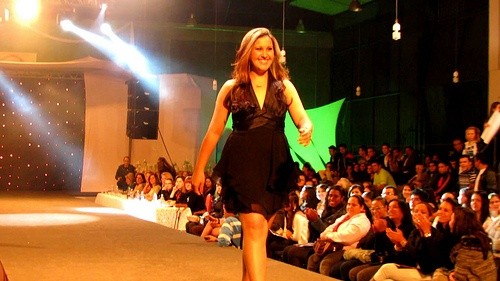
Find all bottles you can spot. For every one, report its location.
[152,193,157,202]
[136,189,139,199]
[130,188,134,198]
[160,193,165,201]
[141,191,144,200]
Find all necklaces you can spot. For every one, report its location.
[256,85,262,87]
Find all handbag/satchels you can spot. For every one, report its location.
[313,240,342,257]
[343,245,378,263]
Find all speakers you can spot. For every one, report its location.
[126,79,160,139]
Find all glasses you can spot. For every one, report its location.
[370,207,385,211]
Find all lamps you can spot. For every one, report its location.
[392,0,402,40]
[55,5,76,26]
[185,9,199,28]
[350,0,363,12]
[295,16,307,34]
[281,0,286,66]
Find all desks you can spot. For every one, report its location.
[95,192,193,231]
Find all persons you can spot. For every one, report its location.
[266,104,500,281]
[115,156,242,248]
[191,28,313,281]
